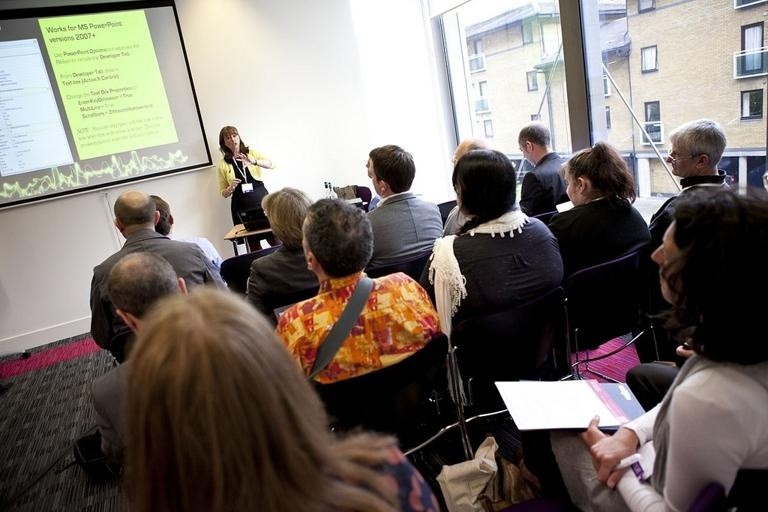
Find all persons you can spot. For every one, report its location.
[493,188,766,511]
[118,283,441,512]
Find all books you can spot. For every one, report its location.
[494,379,647,433]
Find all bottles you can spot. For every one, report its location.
[323,182,338,200]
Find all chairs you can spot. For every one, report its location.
[92,196,768,512]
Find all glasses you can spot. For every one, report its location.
[666,146,703,161]
